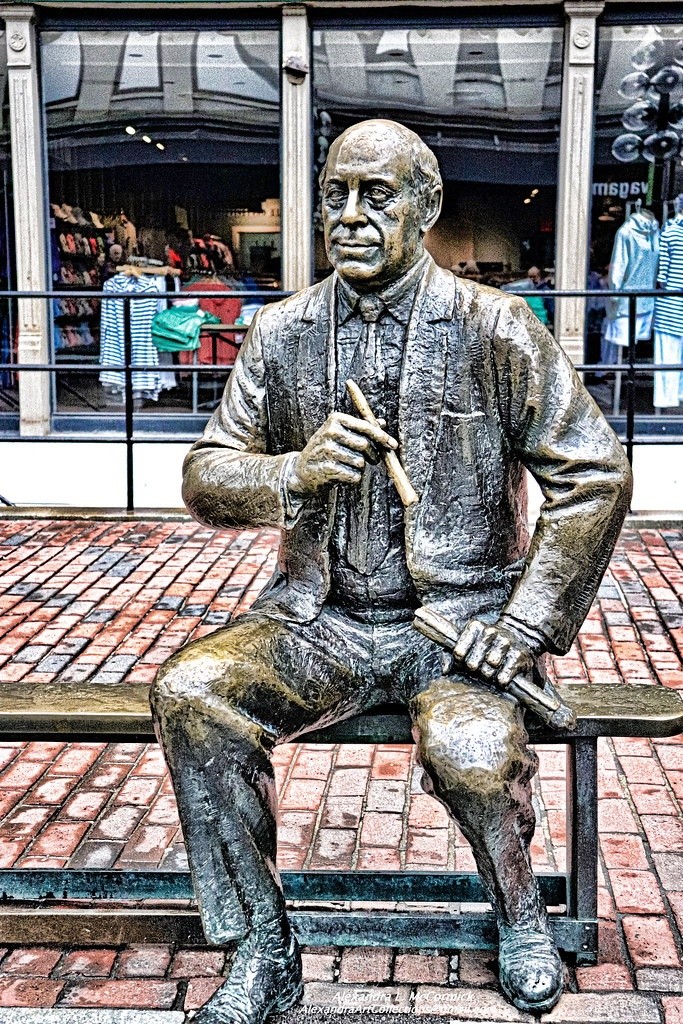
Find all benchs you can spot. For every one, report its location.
[2,682,683,968]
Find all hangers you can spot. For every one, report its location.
[116,253,251,281]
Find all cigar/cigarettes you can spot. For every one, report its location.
[345,378,419,505]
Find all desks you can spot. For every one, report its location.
[192,323,251,414]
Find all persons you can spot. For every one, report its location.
[149,119,633,1024]
